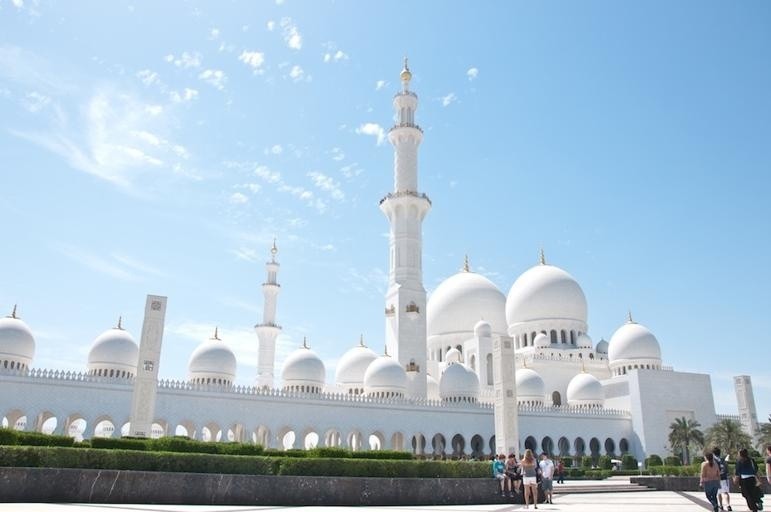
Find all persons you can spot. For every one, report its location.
[612,463,617,470]
[493,449,564,509]
[699,445,771,512]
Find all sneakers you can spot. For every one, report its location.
[545,499,553,504]
[728,505,733,511]
[502,488,523,497]
[720,505,724,511]
